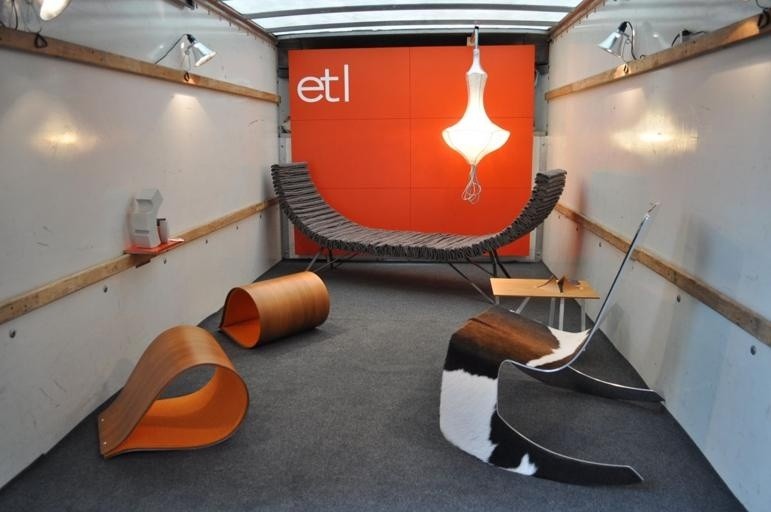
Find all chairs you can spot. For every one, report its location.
[438,202,666,486]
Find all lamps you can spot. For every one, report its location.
[597,21,637,60]
[154,33,217,67]
[26,0,71,21]
[441,25,510,205]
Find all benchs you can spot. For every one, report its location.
[271,160,566,305]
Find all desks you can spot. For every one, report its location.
[488,274,600,332]
